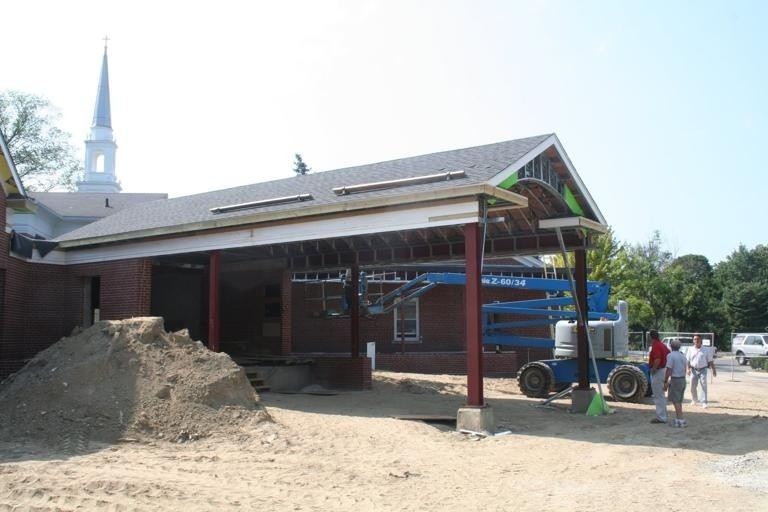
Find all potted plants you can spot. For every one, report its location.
[257,363,312,393]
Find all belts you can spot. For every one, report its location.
[671,377,685,379]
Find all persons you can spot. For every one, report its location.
[685,333,718,411]
[662,338,690,430]
[646,329,671,425]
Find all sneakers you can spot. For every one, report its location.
[671,418,688,432]
[691,399,698,406]
[703,402,709,409]
[650,417,665,424]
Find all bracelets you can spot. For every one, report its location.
[662,380,668,384]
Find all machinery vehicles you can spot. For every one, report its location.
[310,267,651,402]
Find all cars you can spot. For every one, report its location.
[648,336,693,364]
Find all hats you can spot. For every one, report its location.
[671,340,681,349]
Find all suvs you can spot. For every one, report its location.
[730,332,767,365]
